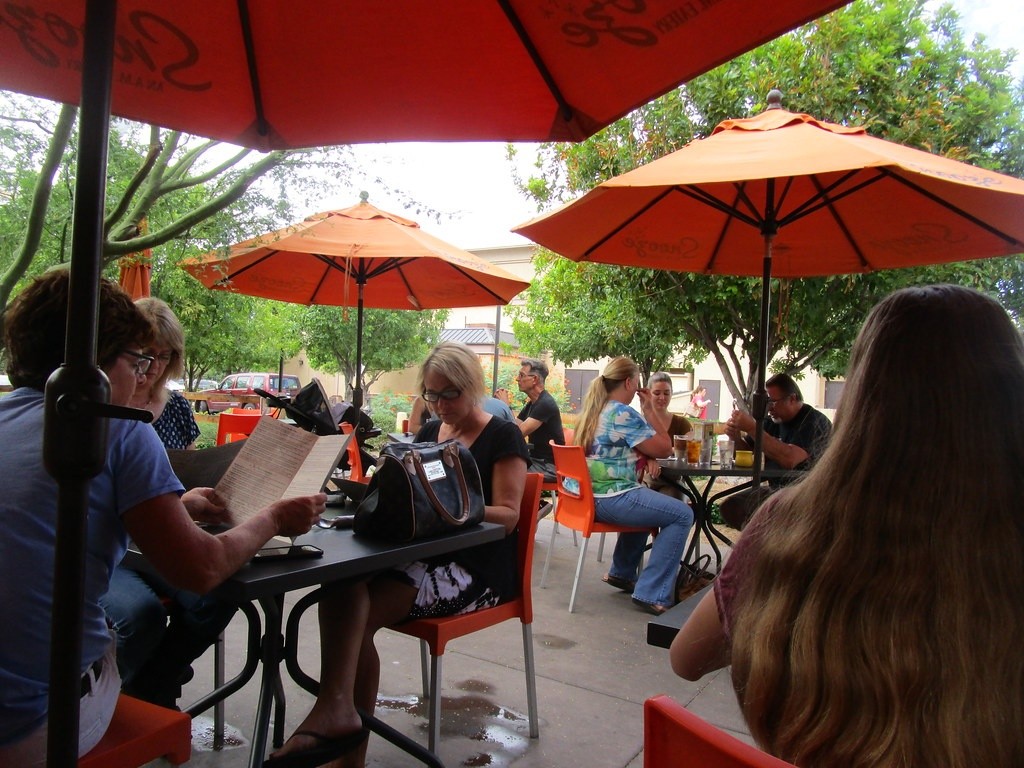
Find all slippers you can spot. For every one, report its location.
[262,730,369,767]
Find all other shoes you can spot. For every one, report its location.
[536,499,552,523]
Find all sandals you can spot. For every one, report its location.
[601,574,636,593]
[631,597,667,616]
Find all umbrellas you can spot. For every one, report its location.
[178,191,530,454]
[118,214,150,302]
[512,91,1024,514]
[0,0,855,768]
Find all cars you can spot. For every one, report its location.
[167,378,218,392]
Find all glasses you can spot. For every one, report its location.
[769,395,790,408]
[143,352,172,365]
[421,388,460,402]
[122,350,155,375]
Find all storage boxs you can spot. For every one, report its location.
[694,422,714,439]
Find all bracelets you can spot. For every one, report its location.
[509,406,512,411]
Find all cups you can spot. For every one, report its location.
[719,440,735,468]
[686,438,702,465]
[736,450,753,467]
[674,435,688,462]
[260,396,271,415]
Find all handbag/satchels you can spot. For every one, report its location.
[674,555,716,604]
[687,402,702,418]
[353,439,485,543]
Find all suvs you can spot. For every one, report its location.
[195,373,300,415]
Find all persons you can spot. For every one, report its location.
[574,357,693,615]
[263,341,533,768]
[0,270,327,768]
[479,394,516,425]
[98,296,240,710]
[493,358,565,525]
[689,385,711,420]
[718,373,832,530]
[639,372,694,504]
[671,282,1024,768]
[408,397,439,435]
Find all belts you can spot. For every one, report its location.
[82,659,103,697]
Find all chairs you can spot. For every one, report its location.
[230,407,279,443]
[540,439,660,615]
[217,413,273,446]
[541,426,579,546]
[339,421,373,485]
[643,693,799,768]
[385,473,544,768]
[78,692,194,768]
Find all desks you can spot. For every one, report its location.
[646,583,716,648]
[656,458,810,604]
[182,495,507,767]
[387,433,417,444]
